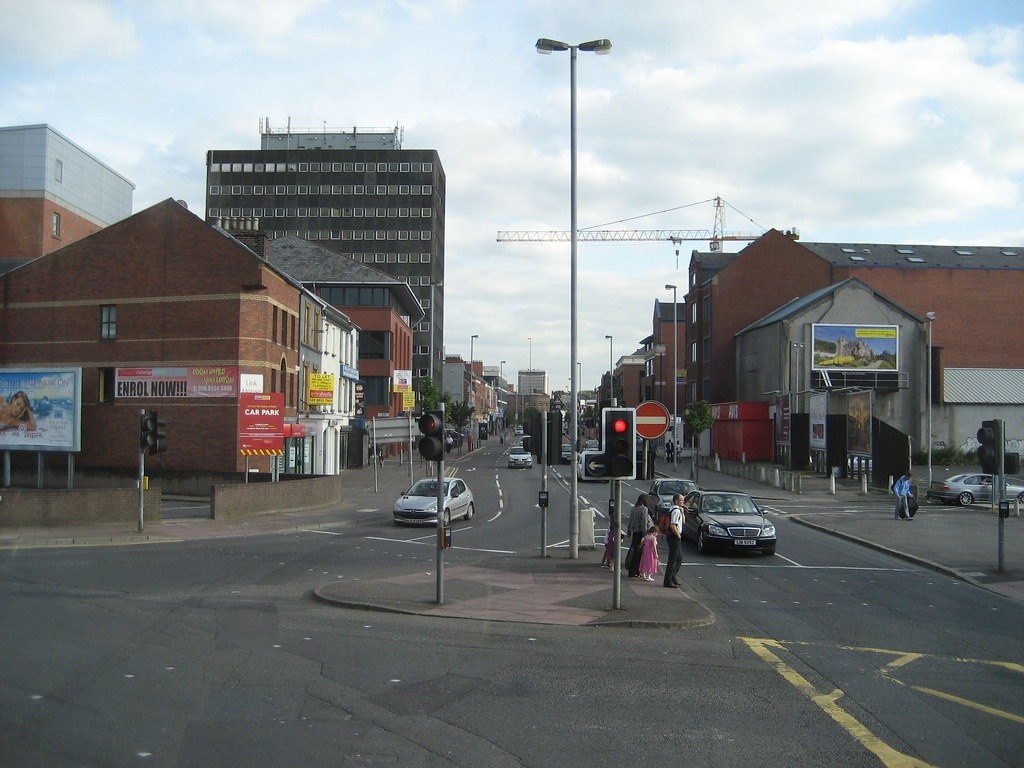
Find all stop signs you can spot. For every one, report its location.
[635,400,671,440]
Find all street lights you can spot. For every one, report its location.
[470,335,478,407]
[577,362,582,396]
[605,335,613,407]
[665,284,677,473]
[501,361,506,409]
[534,38,613,559]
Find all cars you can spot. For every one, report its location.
[392,477,475,528]
[927,473,1024,507]
[514,426,524,435]
[646,478,699,525]
[505,435,531,468]
[562,439,609,482]
[682,487,778,556]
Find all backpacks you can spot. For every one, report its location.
[659,508,680,535]
[890,478,902,493]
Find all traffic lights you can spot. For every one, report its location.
[582,407,636,480]
[976,420,998,466]
[149,410,167,455]
[418,410,444,461]
[140,414,155,450]
[521,411,540,453]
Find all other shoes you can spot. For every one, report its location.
[663,581,678,588]
[672,578,681,585]
[641,576,647,580]
[647,577,654,582]
[628,571,642,578]
[906,517,914,521]
[600,564,605,567]
[895,517,902,520]
[607,563,611,567]
[610,567,622,572]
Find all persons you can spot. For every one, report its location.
[640,527,660,581]
[675,482,689,494]
[981,477,992,484]
[600,524,624,571]
[725,498,744,512]
[650,440,658,460]
[0,390,37,432]
[666,439,673,463]
[663,493,685,588]
[676,441,681,463]
[627,494,648,577]
[445,435,453,454]
[377,446,384,468]
[894,473,914,521]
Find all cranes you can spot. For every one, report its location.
[496,196,799,253]
